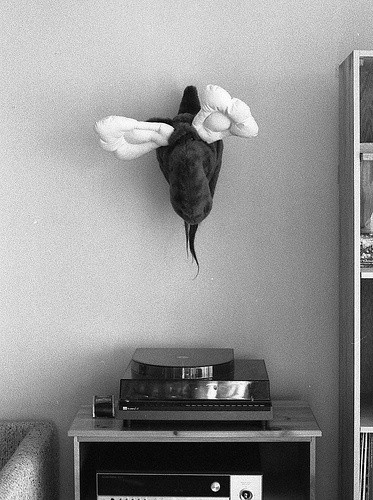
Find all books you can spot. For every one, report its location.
[358,432,373,500]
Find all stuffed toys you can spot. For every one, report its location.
[92,80,263,228]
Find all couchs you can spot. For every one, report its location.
[0,417,61,500]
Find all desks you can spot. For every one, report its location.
[67,399,323,500]
